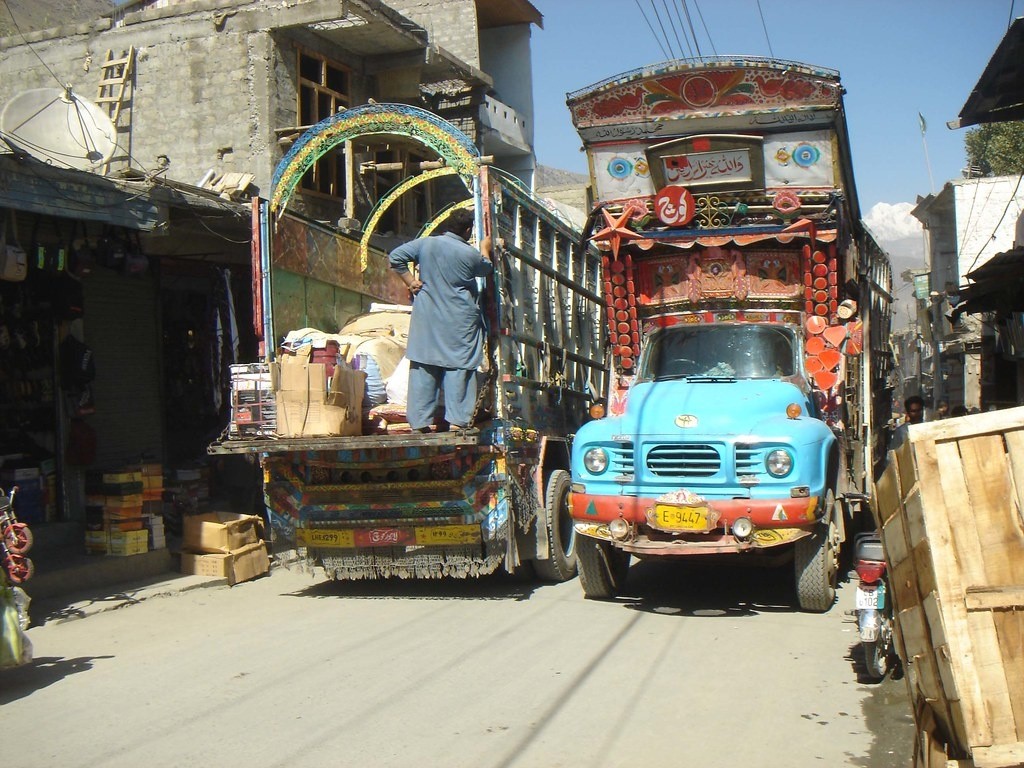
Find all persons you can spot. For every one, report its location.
[889,395,969,449]
[389,208,493,434]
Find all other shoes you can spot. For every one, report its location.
[449,424,461,432]
[410,427,431,435]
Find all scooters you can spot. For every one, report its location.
[836,490,897,679]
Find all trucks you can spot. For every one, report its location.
[565,318,897,613]
[204,239,605,584]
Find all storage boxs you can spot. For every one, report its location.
[182,510,265,555]
[180,539,271,588]
[269,343,368,437]
[84,464,168,559]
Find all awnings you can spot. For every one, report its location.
[946,17,1024,130]
